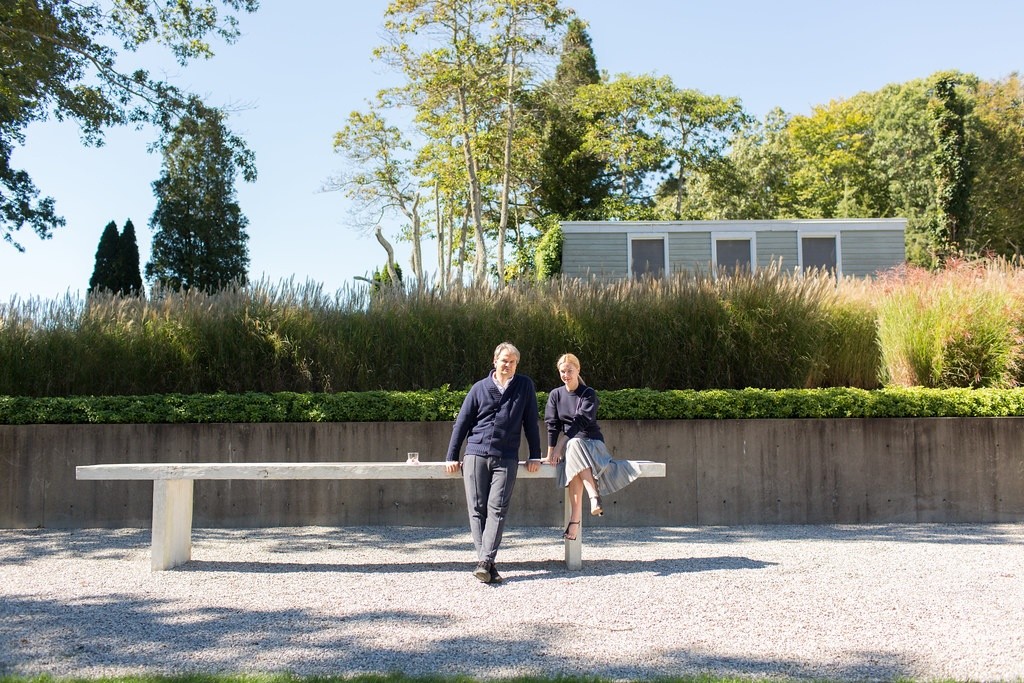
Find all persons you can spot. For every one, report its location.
[446,342,542,584]
[543,353,606,541]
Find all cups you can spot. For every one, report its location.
[407,453,419,464]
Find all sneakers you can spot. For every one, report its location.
[473,561,502,582]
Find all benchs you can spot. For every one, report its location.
[74,460,666,571]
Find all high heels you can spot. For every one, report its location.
[564,521,580,540]
[589,496,604,516]
